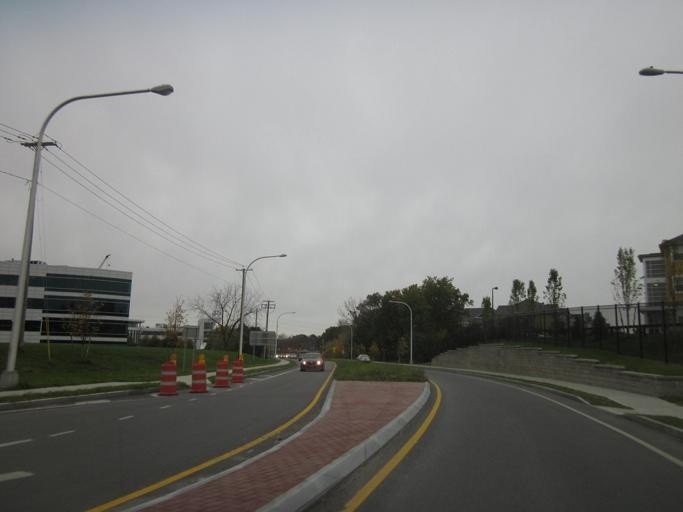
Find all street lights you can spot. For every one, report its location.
[274,310,296,357]
[491,287,498,311]
[638,65,683,77]
[236,253,287,360]
[345,324,352,360]
[0,82,173,391]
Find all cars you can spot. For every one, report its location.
[356,354,371,363]
[388,299,413,364]
[275,350,325,372]
